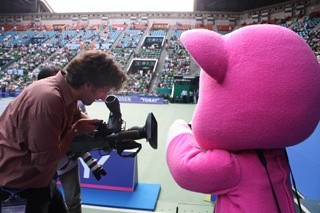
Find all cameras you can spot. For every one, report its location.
[81,152,107,181]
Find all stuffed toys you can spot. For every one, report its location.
[166,23,320,213]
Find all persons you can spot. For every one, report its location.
[38,66,89,213]
[0,15,320,98]
[0,50,127,213]
